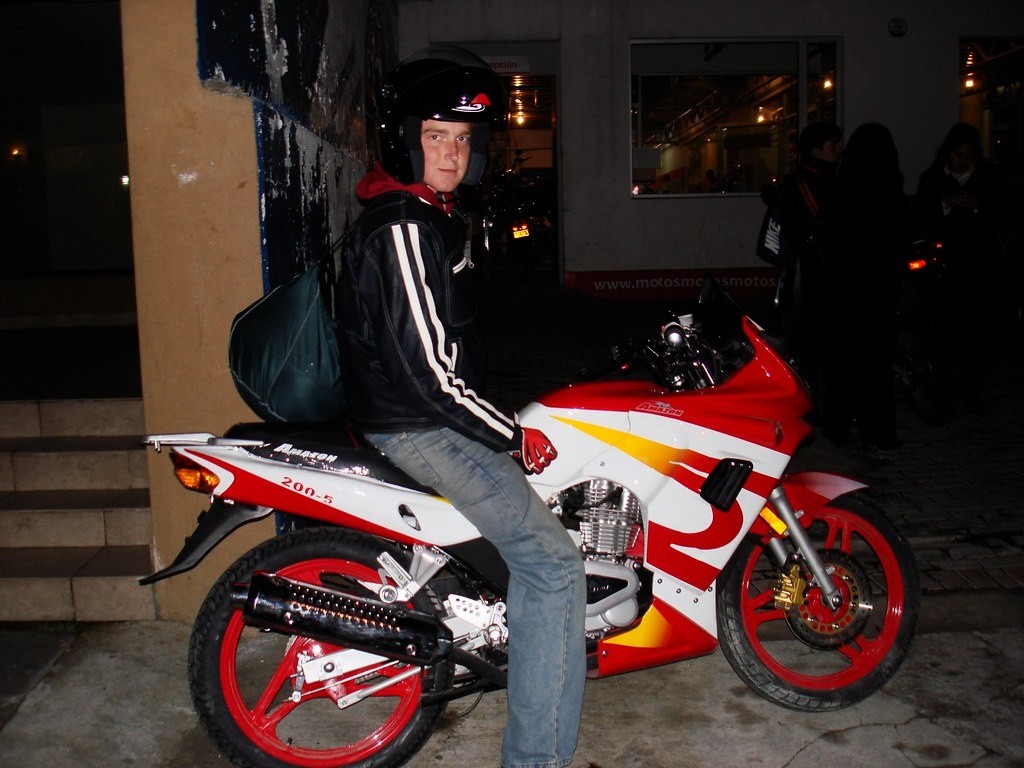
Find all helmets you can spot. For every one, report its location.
[368,44,512,188]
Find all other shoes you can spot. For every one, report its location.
[820,430,849,449]
[860,436,903,452]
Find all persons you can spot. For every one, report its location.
[333,73,587,768]
[661,121,1002,451]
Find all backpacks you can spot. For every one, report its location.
[223,198,474,434]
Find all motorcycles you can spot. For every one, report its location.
[469,147,559,271]
[140,265,925,768]
[893,239,965,431]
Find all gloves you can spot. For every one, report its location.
[506,425,559,474]
